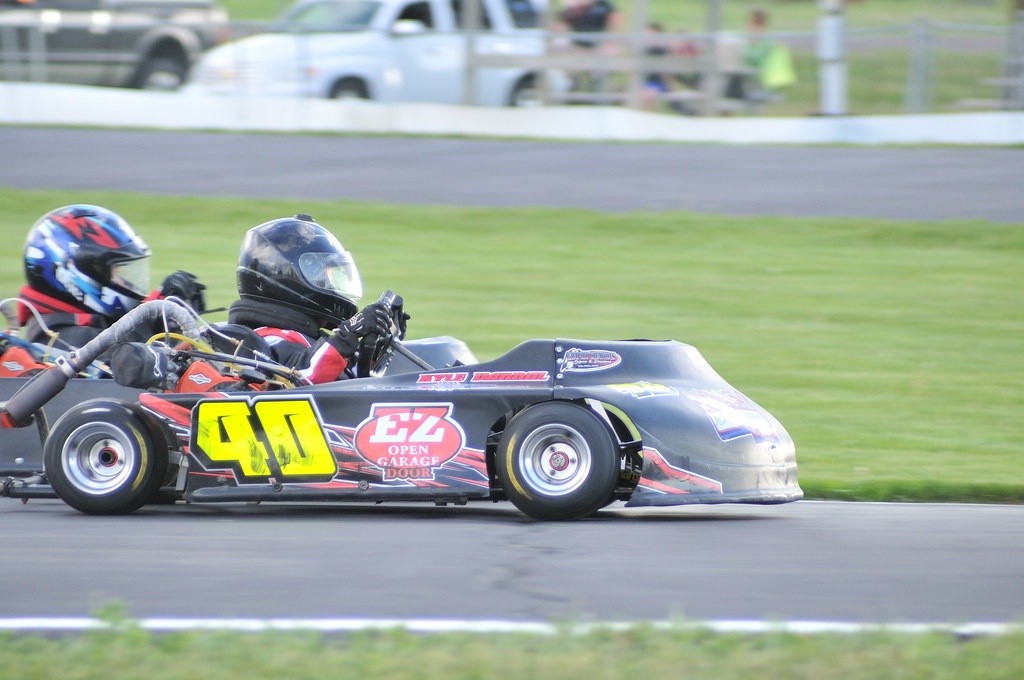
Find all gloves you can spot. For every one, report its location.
[160,269,207,315]
[327,304,412,364]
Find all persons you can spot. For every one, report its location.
[556,0,795,110]
[25,204,411,386]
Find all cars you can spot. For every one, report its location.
[178,0,574,109]
[0,0,230,92]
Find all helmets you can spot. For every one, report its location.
[22,204,153,321]
[238,214,358,323]
